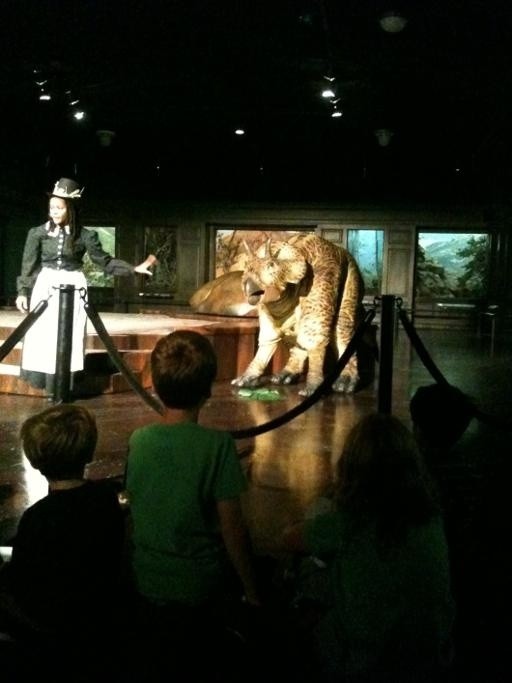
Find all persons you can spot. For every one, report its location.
[14,177,157,407]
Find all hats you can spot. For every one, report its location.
[46,176,86,202]
